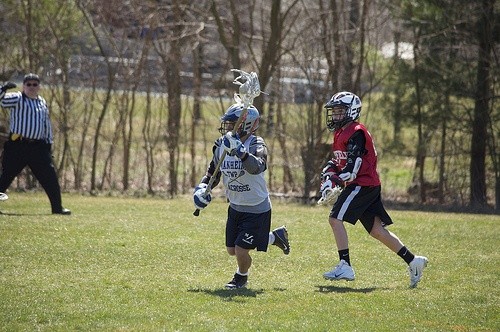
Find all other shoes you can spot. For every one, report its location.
[53,208,71,215]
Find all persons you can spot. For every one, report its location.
[317,89,430,288]
[0,73,70,216]
[192,102,291,291]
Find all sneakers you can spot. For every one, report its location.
[409,255,428,289]
[223,267,249,289]
[322,260,355,280]
[272,225,291,255]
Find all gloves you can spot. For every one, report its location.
[223,130,247,158]
[4,80,17,88]
[193,185,212,210]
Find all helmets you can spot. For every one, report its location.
[323,92,362,132]
[218,103,260,139]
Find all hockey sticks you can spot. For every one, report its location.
[316,185,343,206]
[192,69,261,216]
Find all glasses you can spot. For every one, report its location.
[24,83,40,88]
